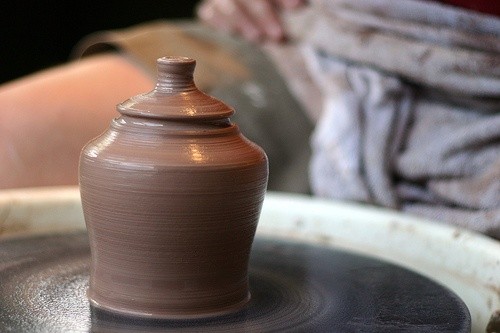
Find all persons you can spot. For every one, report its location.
[1,1,500,238]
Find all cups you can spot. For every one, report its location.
[78,54,269,322]
